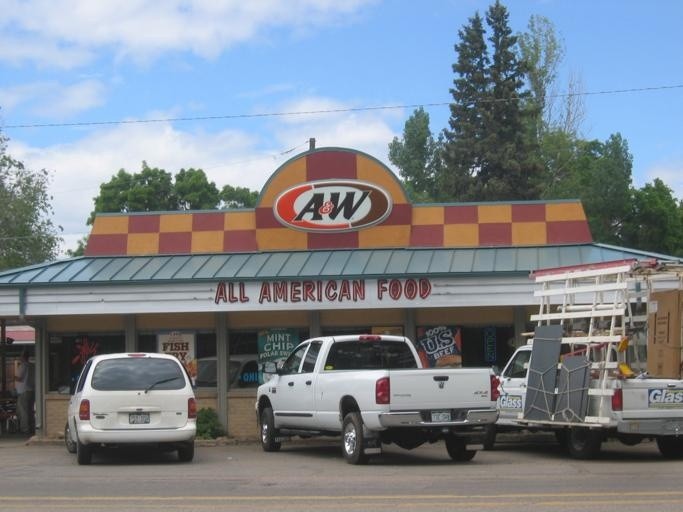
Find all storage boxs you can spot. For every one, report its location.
[646,289,683,379]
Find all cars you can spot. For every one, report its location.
[195,352,259,388]
[63,350,198,465]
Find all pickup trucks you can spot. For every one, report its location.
[252,332,503,466]
[495,338,683,464]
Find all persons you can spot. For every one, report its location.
[12,350,34,438]
